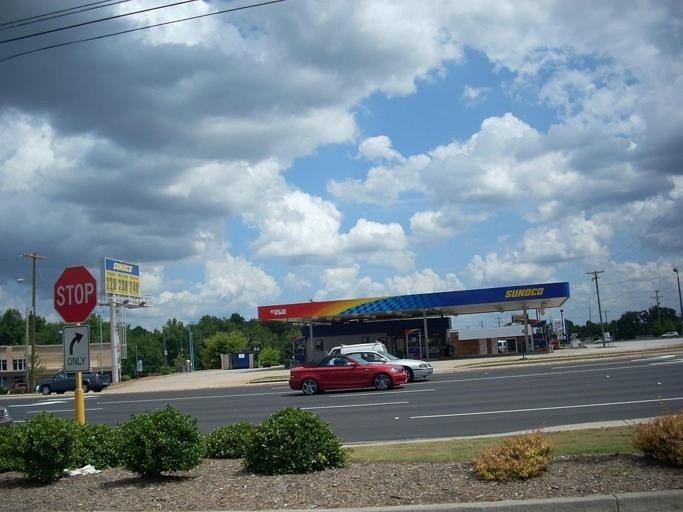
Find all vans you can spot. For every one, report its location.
[328,341,388,355]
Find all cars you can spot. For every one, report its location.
[662,332,679,338]
[0,404,12,423]
[289,351,433,395]
[498,340,506,352]
[594,338,612,344]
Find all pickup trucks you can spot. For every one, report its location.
[38,368,110,395]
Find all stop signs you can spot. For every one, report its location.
[54,265,97,323]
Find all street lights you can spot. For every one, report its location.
[672,267,683,320]
[17,277,30,392]
